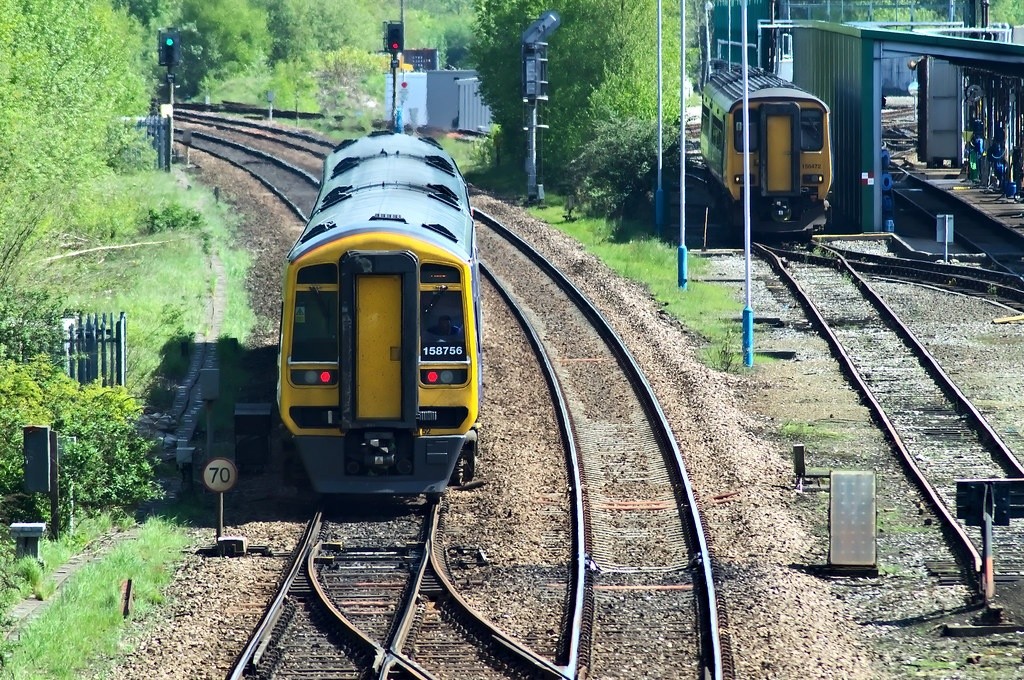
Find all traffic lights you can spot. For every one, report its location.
[388,23,404,53]
[162,32,179,66]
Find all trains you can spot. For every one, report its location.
[700,65,833,231]
[275,130,482,497]
